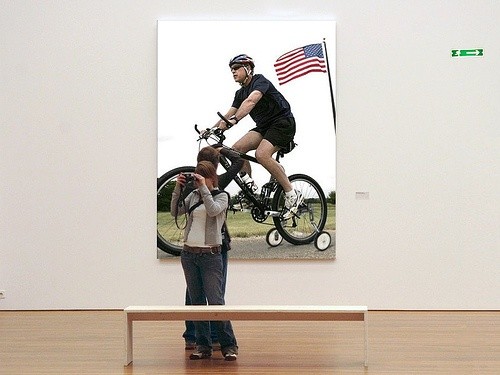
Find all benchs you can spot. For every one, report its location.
[123,306,370,368]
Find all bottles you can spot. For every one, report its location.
[240,171,259,194]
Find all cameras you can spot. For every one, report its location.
[180,172,195,186]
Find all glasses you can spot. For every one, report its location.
[231,65,249,71]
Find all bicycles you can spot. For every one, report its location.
[156,112,332,258]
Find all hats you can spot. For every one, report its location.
[228,53,255,69]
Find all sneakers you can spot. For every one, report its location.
[280,189,304,219]
[228,186,258,210]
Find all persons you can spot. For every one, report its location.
[182,147,245,351]
[170,161,239,361]
[200,54,305,221]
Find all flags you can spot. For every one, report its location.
[274,43,326,85]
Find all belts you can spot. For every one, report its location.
[182,244,221,254]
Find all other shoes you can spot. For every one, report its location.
[212,343,222,351]
[224,353,237,360]
[189,352,210,360]
[185,341,194,350]
